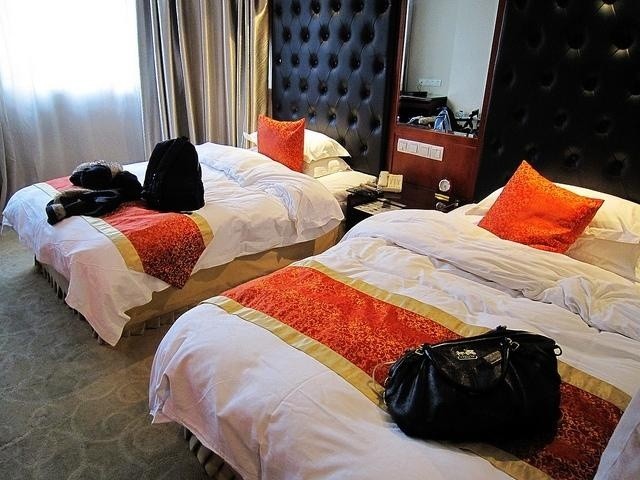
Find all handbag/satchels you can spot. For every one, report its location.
[383,325,563,457]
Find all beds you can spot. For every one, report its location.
[0,147,378,348]
[149,203,640,480]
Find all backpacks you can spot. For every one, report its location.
[433,105,470,134]
[141,136,205,215]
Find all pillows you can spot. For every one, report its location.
[258,113,305,173]
[468,180,640,281]
[303,156,354,178]
[243,128,352,163]
[477,158,604,255]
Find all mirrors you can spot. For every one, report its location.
[396,0,498,140]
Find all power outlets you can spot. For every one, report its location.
[416,78,441,89]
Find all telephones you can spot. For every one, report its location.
[377,171,403,193]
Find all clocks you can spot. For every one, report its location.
[432,175,456,206]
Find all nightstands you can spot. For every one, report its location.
[345,181,463,234]
